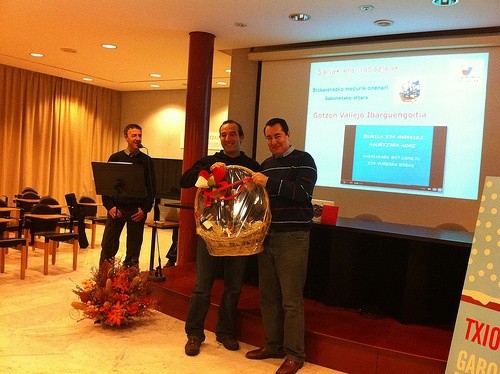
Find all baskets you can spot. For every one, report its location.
[194,166,272,256]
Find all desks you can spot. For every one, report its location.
[215,217,474,332]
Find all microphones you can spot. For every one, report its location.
[137,143,147,149]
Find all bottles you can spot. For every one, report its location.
[194,211,218,239]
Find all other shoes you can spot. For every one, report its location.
[163,259,174,268]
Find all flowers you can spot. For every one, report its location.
[71,256,160,332]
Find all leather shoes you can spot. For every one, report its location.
[185,338,200,356]
[275,360,304,374]
[246,348,285,359]
[216,336,239,350]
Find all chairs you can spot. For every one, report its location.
[353,214,381,222]
[435,223,468,232]
[0,187,107,280]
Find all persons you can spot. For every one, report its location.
[180,120,260,357]
[244,117,317,374]
[99,123,156,267]
[163,227,178,266]
